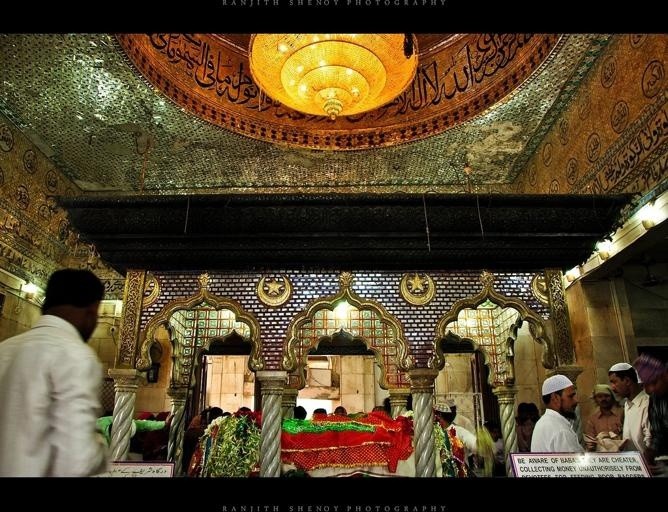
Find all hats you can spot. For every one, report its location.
[439,398,456,409]
[433,402,451,413]
[608,362,633,373]
[541,374,573,397]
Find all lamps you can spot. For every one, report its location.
[248,34,418,120]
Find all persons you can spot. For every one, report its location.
[181,395,505,478]
[515,353,667,476]
[0,269,110,477]
